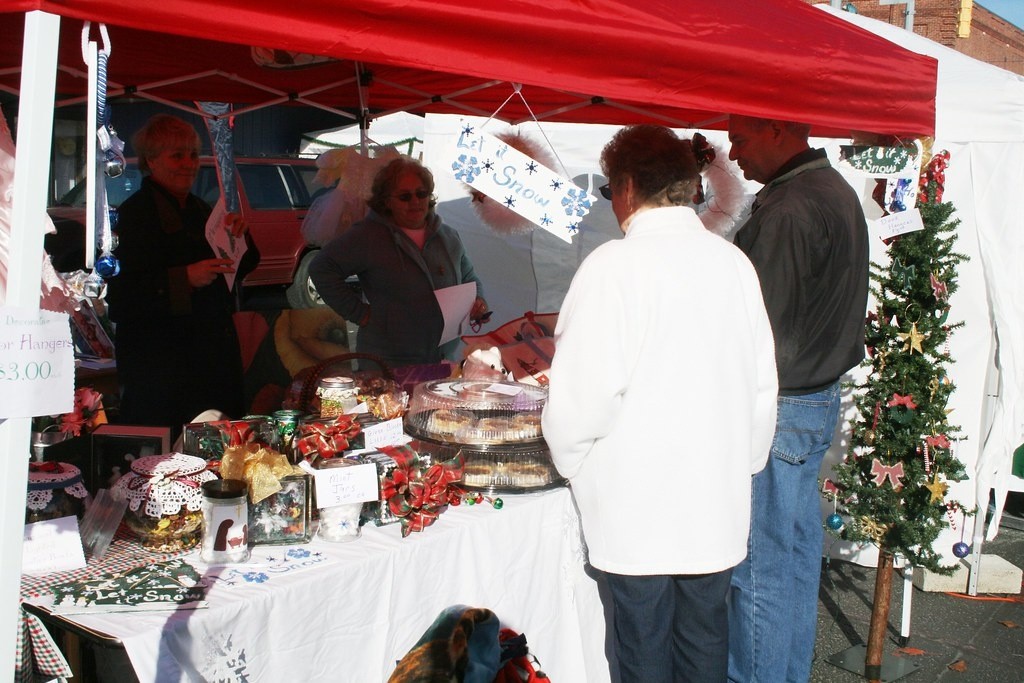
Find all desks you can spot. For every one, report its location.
[22,487,622,683]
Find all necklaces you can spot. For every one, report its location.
[406,226,445,276]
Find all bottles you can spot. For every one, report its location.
[312,457,362,540]
[316,377,358,416]
[200,479,250,563]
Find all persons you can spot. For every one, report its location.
[306,158,491,371]
[540,124,780,683]
[104,112,262,452]
[727,108,870,683]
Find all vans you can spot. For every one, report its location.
[46,156,363,313]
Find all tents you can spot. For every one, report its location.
[0,0,939,648]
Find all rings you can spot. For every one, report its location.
[482,305,485,310]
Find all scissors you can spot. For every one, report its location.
[469,311,494,333]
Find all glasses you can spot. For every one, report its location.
[598,184,612,200]
[390,190,430,200]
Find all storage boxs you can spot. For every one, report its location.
[179,417,313,545]
